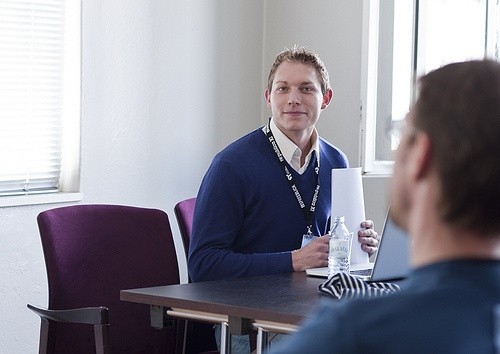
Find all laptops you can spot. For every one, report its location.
[306,205,414,282]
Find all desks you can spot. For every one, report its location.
[120,271,335,354]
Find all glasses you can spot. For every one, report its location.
[386,121,420,151]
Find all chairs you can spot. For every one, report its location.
[28,197,220,354]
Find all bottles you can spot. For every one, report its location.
[328,216,350,280]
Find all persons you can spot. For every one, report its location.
[269,59,500,354]
[188,50,378,283]
